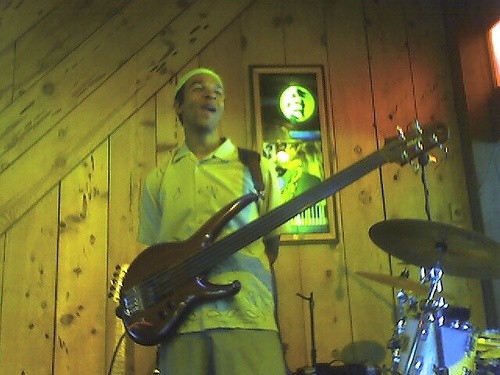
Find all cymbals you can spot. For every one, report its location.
[368,219,500,276]
[354,268,454,300]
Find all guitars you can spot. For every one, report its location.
[106,118,449,346]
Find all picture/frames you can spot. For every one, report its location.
[247,63,339,246]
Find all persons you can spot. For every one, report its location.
[133,67,290,375]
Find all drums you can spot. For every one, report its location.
[390,318,475,375]
[392,303,475,375]
